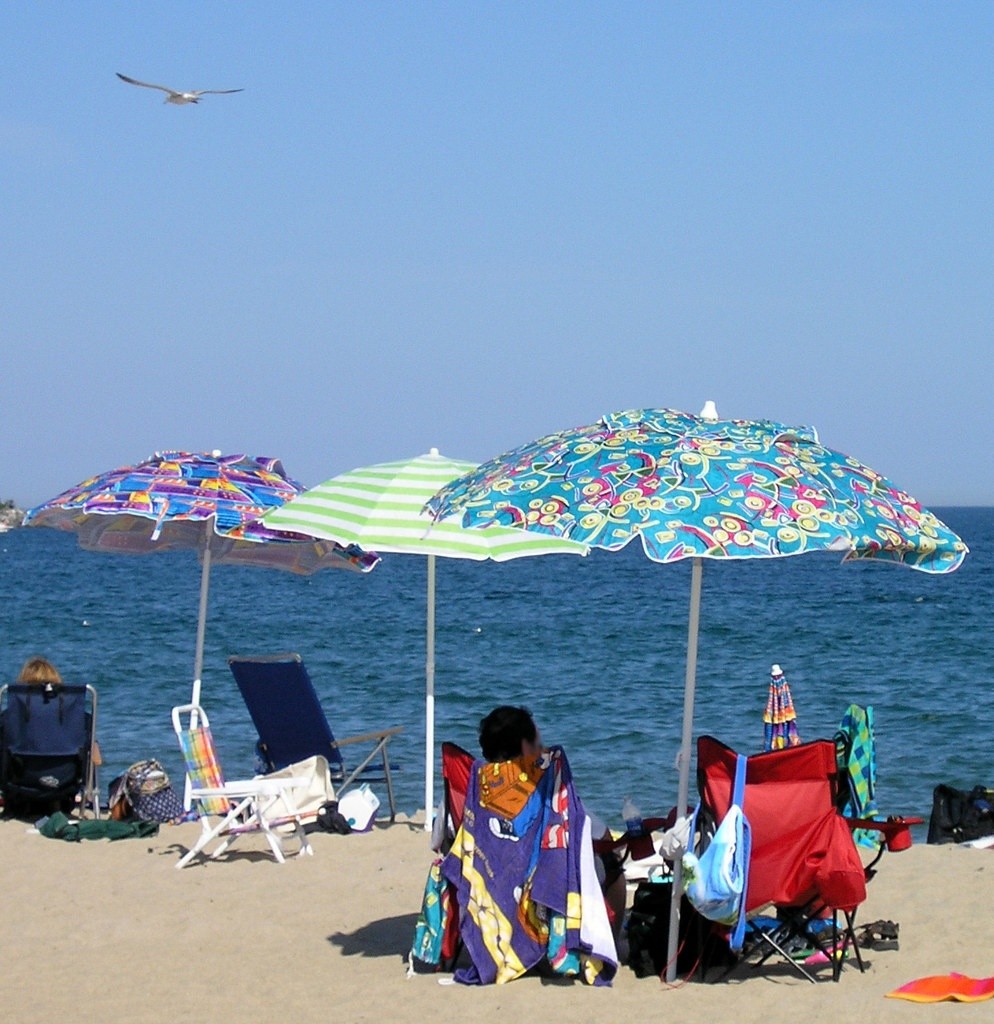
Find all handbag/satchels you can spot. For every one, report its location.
[927,784,994,845]
[107,758,183,825]
[887,814,924,852]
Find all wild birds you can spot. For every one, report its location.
[115,71,245,106]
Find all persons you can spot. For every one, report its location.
[0,657,105,770]
[479,704,628,948]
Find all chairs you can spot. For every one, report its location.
[172,704,314,870]
[668,730,923,983]
[227,654,404,824]
[0,682,100,821]
[440,739,665,971]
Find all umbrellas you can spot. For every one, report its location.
[19,450,384,821]
[760,662,808,756]
[416,395,972,985]
[252,444,594,850]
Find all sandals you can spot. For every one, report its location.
[854,919,900,950]
[817,925,846,949]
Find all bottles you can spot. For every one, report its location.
[621,796,645,836]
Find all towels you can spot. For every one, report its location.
[837,703,885,851]
[440,745,618,987]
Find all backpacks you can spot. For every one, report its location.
[685,755,751,950]
